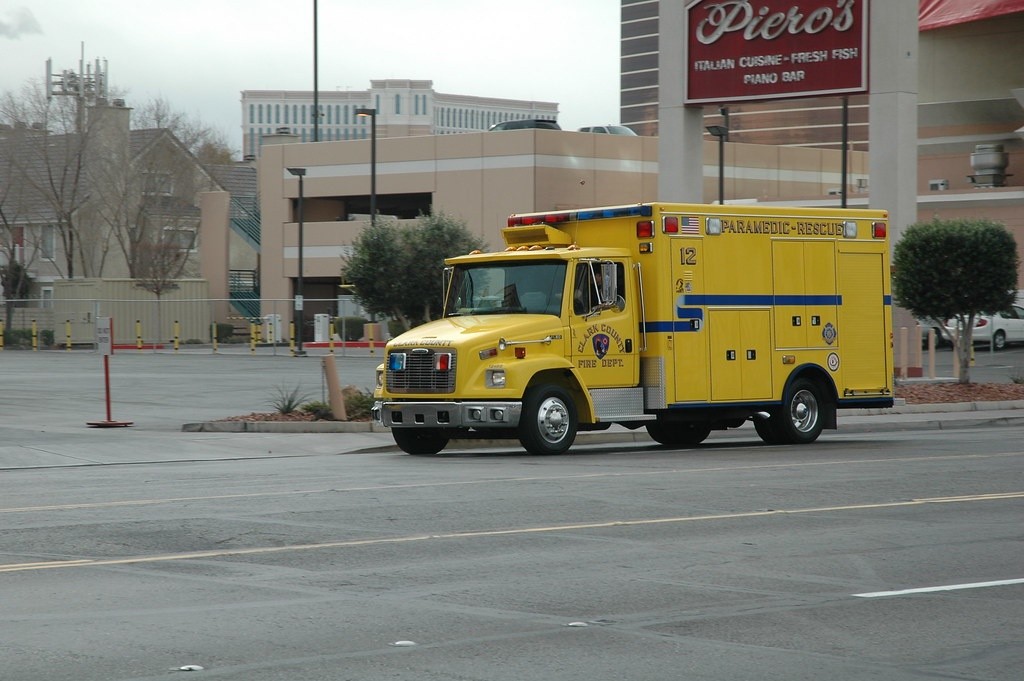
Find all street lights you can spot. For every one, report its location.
[354,107,380,322]
[286,166,308,356]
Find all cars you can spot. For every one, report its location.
[941,303,1024,349]
[916,313,949,349]
[577,123,640,136]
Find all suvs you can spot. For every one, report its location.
[487,118,562,130]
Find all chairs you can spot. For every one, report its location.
[519,291,586,315]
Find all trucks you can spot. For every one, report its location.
[369,202,896,454]
